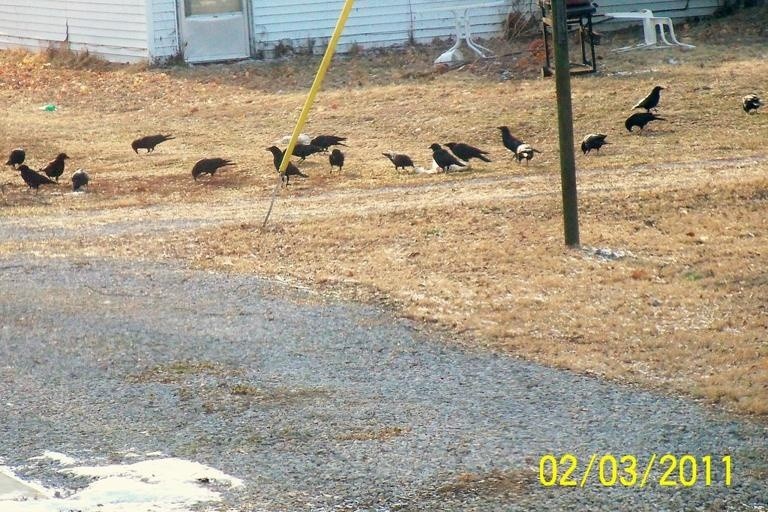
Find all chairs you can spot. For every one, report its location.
[536,0,599,76]
[605,8,697,55]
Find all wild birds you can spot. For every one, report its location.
[427,140,491,176]
[739,91,763,116]
[497,123,542,167]
[380,152,416,174]
[4,148,88,196]
[191,156,237,181]
[623,84,668,134]
[266,133,350,186]
[131,133,178,155]
[580,132,615,157]
